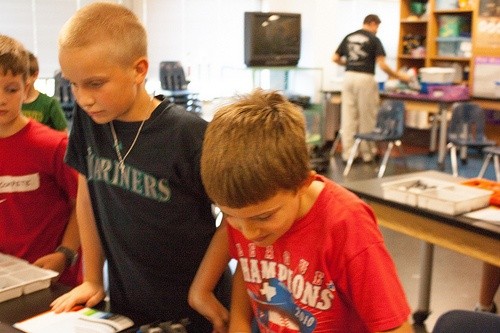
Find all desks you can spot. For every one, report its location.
[322,90,500,173]
[340,169,500,323]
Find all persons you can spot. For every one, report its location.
[21,53,67,133]
[334,14,412,165]
[201,86,415,333]
[0,34,84,288]
[50,1,233,314]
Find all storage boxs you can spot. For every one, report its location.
[428,86,469,100]
[1,253,59,305]
[435,37,471,57]
[419,67,455,84]
[381,176,494,216]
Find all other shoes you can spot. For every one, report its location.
[363,156,382,165]
[342,157,364,166]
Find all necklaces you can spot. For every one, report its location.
[110,96,155,175]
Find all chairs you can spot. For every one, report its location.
[154,62,202,116]
[445,103,500,183]
[53,72,76,120]
[343,99,408,179]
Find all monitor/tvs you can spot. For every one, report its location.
[244,12,301,67]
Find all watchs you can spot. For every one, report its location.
[55,244,79,269]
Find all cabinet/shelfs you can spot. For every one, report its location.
[252,66,326,173]
[397,0,500,84]
